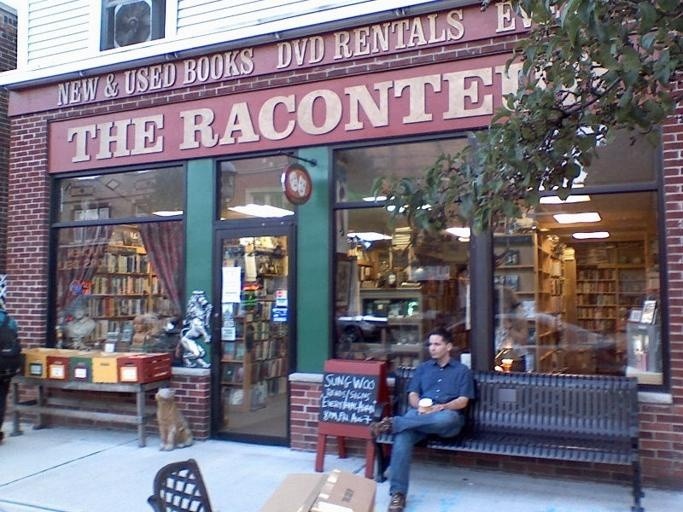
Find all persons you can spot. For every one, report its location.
[368,327,475,512]
[182,310,212,368]
[62,297,95,338]
[0,299,23,442]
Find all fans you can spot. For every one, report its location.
[114,0,160,48]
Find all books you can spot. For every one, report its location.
[221,300,287,410]
[494,249,656,375]
[86,253,174,346]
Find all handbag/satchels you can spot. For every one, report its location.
[0,315,25,377]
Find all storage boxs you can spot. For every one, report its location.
[20,348,173,384]
[257,469,377,512]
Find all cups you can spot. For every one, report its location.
[419,398,432,413]
[387,272,397,287]
[501,359,513,372]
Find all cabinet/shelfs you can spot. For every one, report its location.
[85,244,287,413]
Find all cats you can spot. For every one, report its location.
[155,386,193,451]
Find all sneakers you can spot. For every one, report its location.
[368,416,391,435]
[388,494,405,512]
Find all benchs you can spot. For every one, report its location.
[10,371,171,448]
[372,366,644,512]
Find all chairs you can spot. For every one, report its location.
[147,458,213,512]
